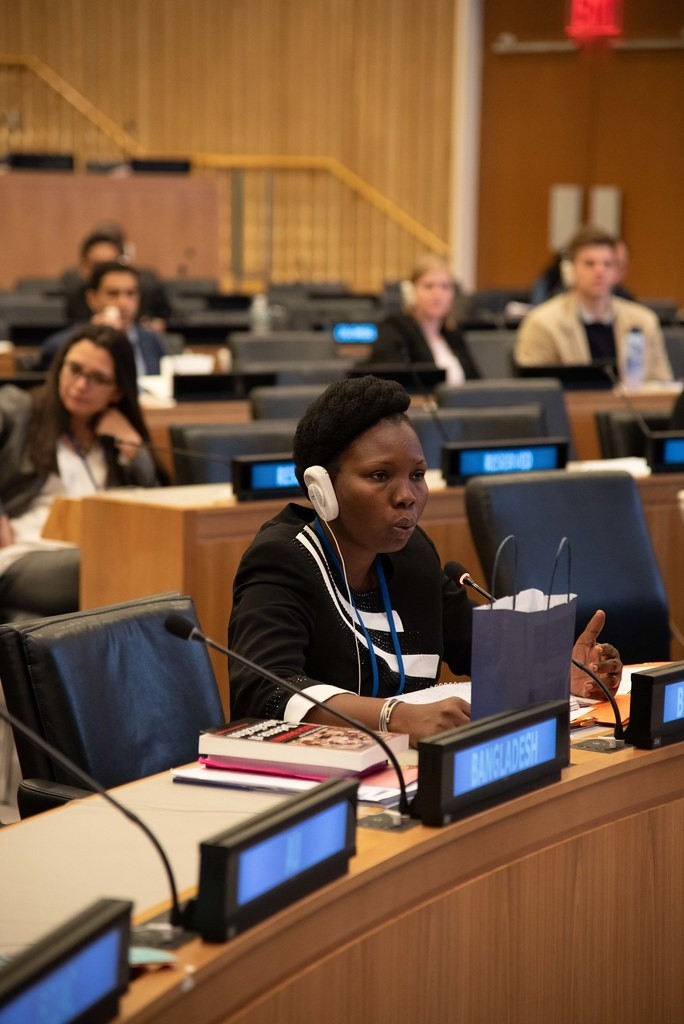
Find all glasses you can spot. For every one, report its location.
[61,357,117,389]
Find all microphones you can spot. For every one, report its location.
[164,611,422,835]
[400,347,452,444]
[599,355,651,434]
[445,559,626,751]
[98,434,232,466]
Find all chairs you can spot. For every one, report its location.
[434,380,579,461]
[465,470,673,667]
[230,333,337,366]
[595,407,673,471]
[248,385,329,422]
[0,276,540,344]
[0,592,226,822]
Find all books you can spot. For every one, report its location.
[385,681,596,732]
[198,715,408,784]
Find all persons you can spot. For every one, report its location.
[42,263,169,376]
[0,322,162,613]
[531,248,573,305]
[227,377,623,747]
[371,255,484,381]
[64,232,172,318]
[610,240,636,299]
[516,226,675,382]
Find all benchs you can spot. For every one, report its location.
[170,404,546,485]
[469,330,684,380]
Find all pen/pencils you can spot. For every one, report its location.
[570,716,598,728]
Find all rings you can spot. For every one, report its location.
[614,676,619,688]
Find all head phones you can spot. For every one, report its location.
[304,465,340,522]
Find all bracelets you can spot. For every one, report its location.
[378,698,405,731]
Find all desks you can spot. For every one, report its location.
[0,327,684,1024]
